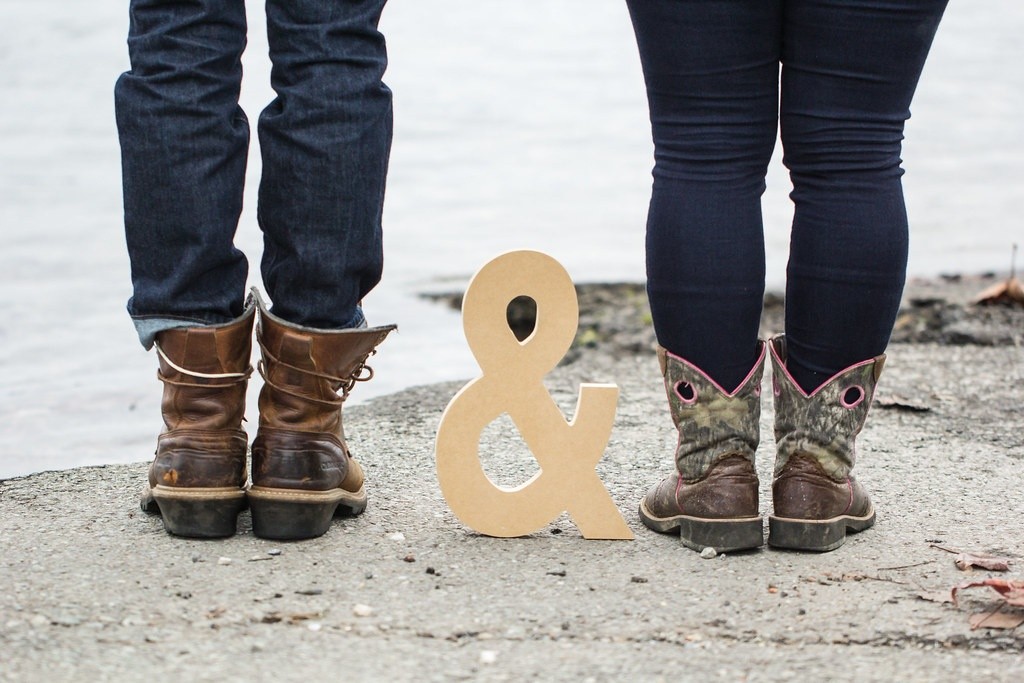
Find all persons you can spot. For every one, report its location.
[114,0,394,541]
[626,0,954,553]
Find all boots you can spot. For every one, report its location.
[246,288,398,539]
[768,332,886,552]
[639,337,766,553]
[141,291,256,538]
